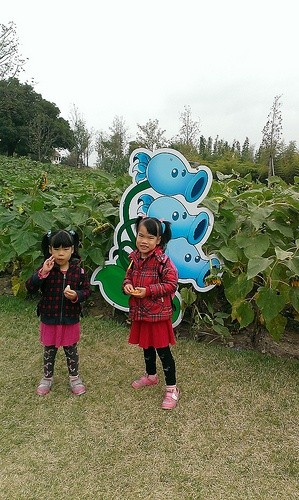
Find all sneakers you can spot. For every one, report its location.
[161,385,181,411]
[36,378,54,396]
[131,374,159,390]
[68,375,86,396]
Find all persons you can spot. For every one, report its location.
[121,215,182,410]
[25,230,91,396]
